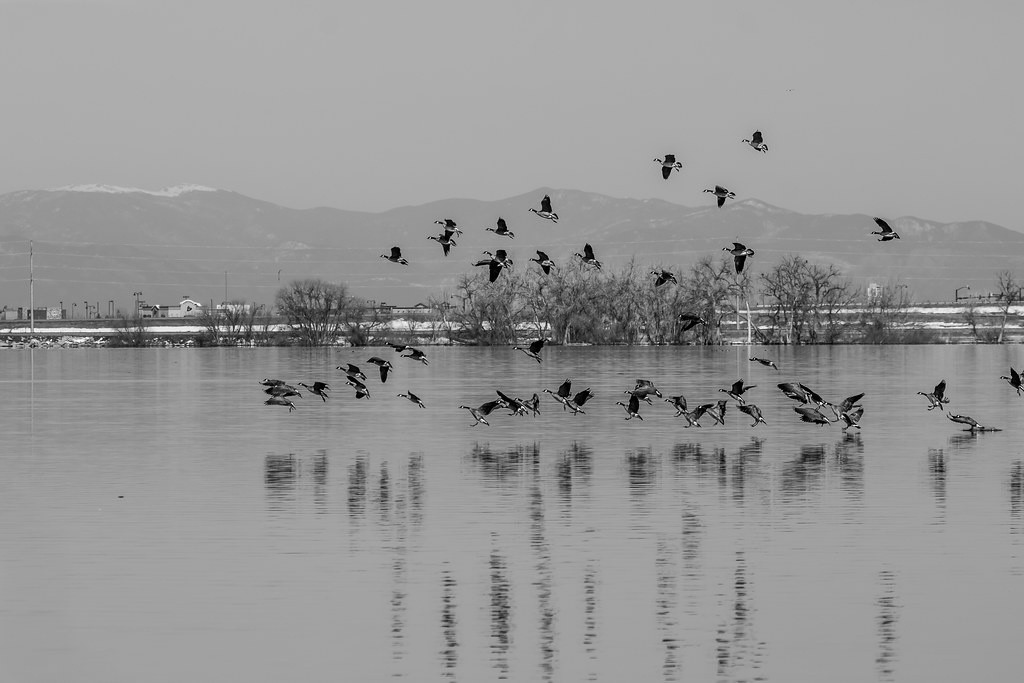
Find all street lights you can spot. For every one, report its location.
[84,301,88,320]
[72,303,77,319]
[450,294,469,327]
[109,300,113,318]
[59,301,62,319]
[895,284,907,310]
[367,300,376,313]
[133,290,144,324]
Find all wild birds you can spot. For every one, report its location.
[379,194,603,286]
[646,129,769,340]
[512,338,550,364]
[916,379,952,411]
[458,355,866,431]
[1000,366,1024,396]
[258,341,430,413]
[869,217,900,241]
[944,411,985,432]
